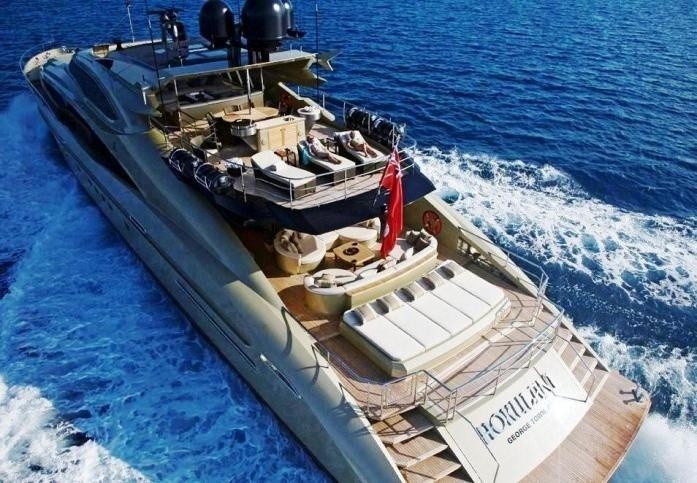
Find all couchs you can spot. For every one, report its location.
[270,215,438,316]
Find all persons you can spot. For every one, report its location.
[347,128,376,158]
[307,132,343,165]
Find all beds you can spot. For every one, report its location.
[338,260,512,377]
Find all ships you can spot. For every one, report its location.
[16,0,651,483]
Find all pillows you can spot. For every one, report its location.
[345,263,463,325]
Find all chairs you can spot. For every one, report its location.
[250,130,392,199]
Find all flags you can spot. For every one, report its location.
[380,147,403,260]
